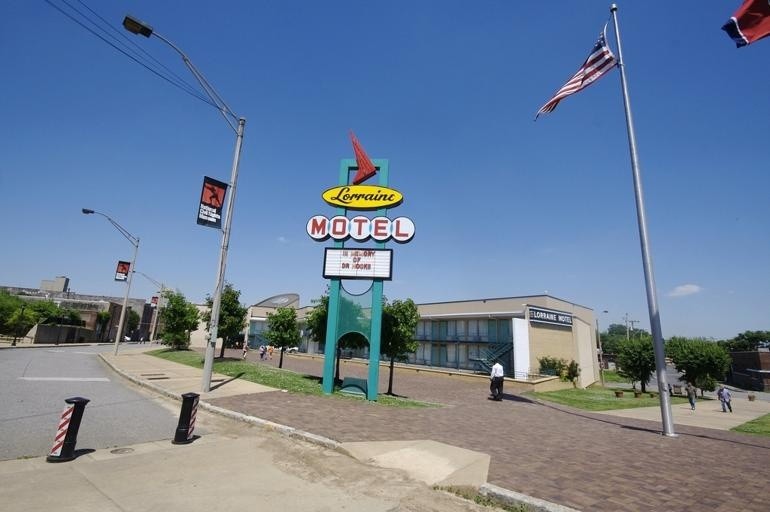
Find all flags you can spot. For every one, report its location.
[720,0,770,50]
[533,14,619,122]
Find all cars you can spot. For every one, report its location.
[121,335,132,343]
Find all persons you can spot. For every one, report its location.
[717,383,733,414]
[242,342,249,359]
[263,345,268,360]
[205,183,221,208]
[684,381,697,409]
[258,345,265,362]
[268,344,274,359]
[487,357,505,401]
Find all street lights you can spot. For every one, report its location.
[55,306,69,346]
[133,269,166,343]
[596,310,610,386]
[12,302,28,347]
[122,13,249,393]
[77,206,142,356]
